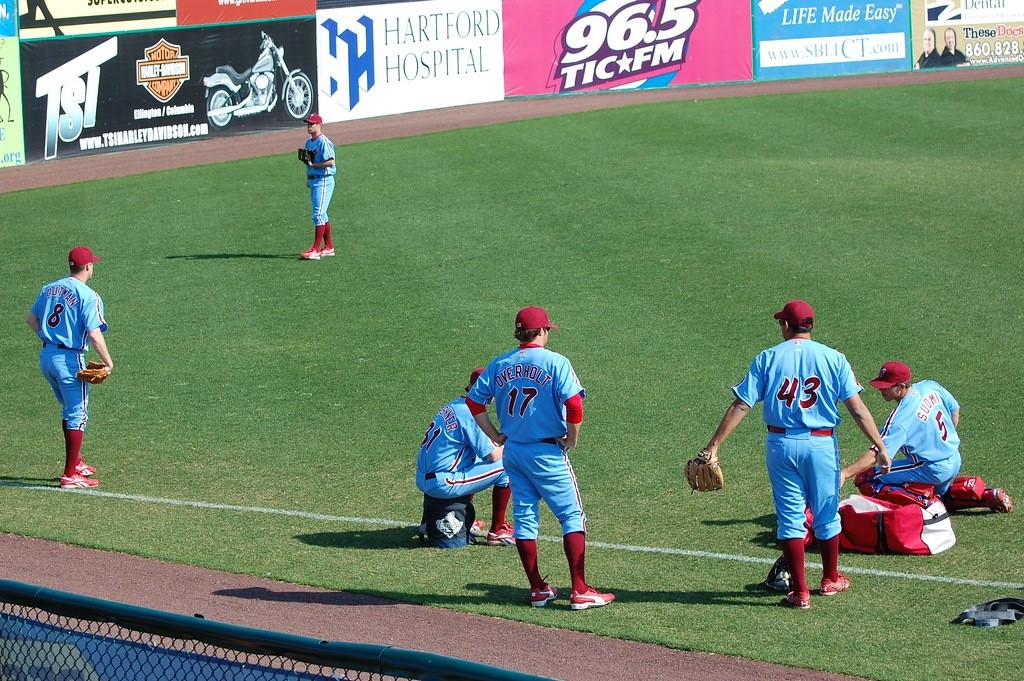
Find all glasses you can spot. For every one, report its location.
[884,384,900,390]
[779,319,790,327]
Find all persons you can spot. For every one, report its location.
[914,28,940,69]
[939,28,971,67]
[838,361,1012,514]
[298,113,337,260]
[683,300,891,609]
[414,367,517,546]
[462,306,616,609]
[25,246,113,488]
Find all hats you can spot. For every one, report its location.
[464,368,484,393]
[773,299,815,328]
[869,360,911,389]
[515,306,558,328]
[69,247,100,267]
[303,113,322,124]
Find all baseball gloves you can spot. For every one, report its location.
[684,449,724,492]
[78,361,110,384]
[297,148,316,164]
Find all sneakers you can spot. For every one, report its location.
[571,585,615,609]
[298,247,321,260]
[819,573,851,595]
[787,591,811,609]
[75,455,96,475]
[319,245,335,257]
[61,471,100,488]
[983,488,1014,514]
[487,521,517,546]
[469,519,485,536]
[764,561,789,590]
[531,575,558,607]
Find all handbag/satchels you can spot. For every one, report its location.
[837,492,957,555]
[420,492,472,549]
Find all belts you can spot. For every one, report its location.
[766,425,834,436]
[307,174,320,180]
[42,342,69,350]
[541,438,557,445]
